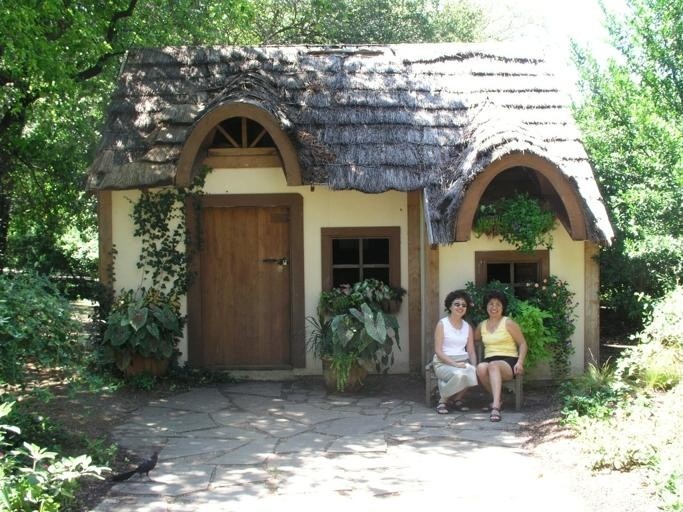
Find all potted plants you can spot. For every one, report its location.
[102,300,181,380]
[300,280,408,392]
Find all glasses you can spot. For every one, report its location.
[453,302,467,307]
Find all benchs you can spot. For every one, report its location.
[423,340,525,411]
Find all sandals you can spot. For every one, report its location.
[451,400,469,412]
[436,402,448,414]
[490,407,501,422]
[482,403,504,412]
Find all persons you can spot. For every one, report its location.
[433,290,478,415]
[473,288,528,422]
